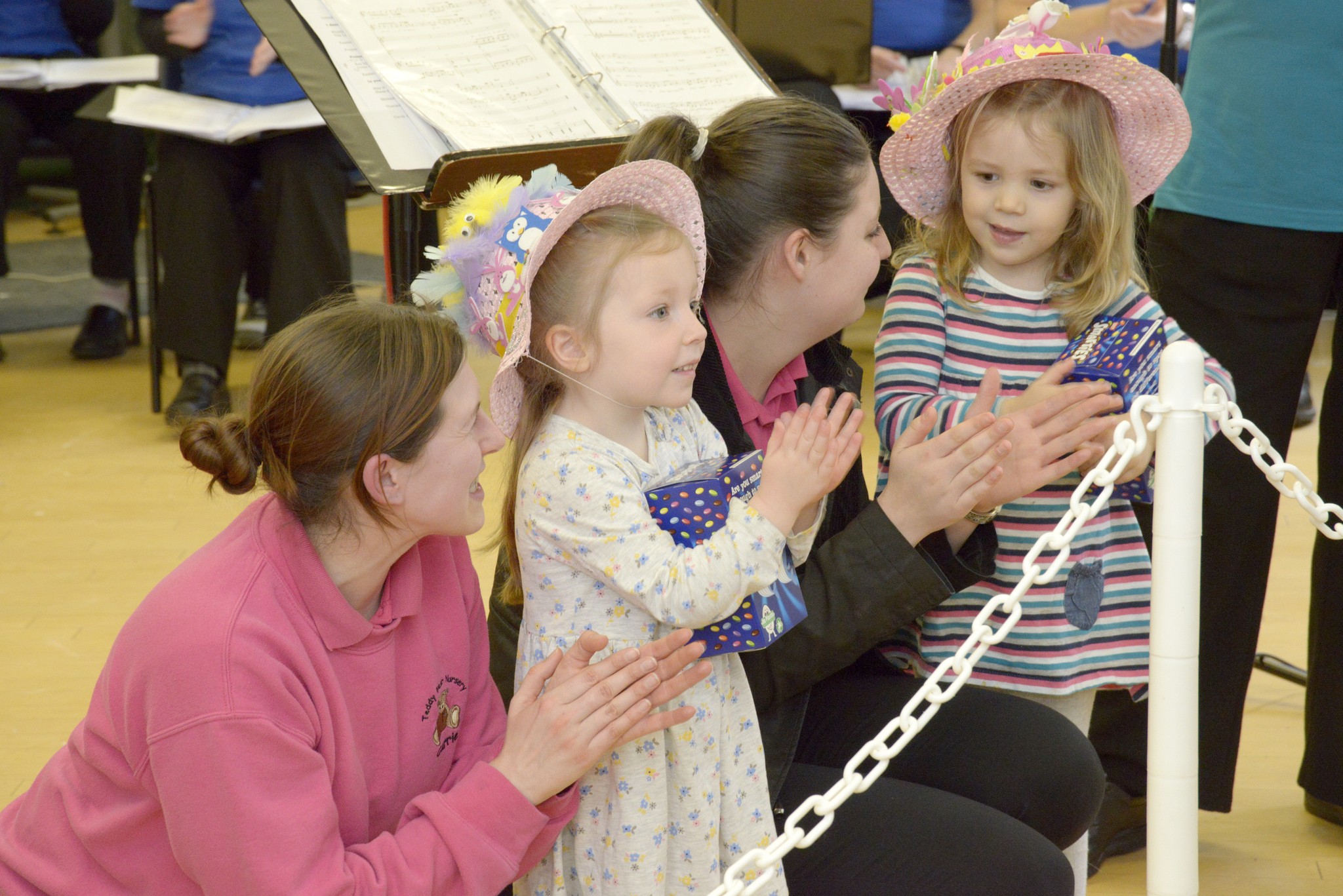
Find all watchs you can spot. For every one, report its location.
[964,505,1002,524]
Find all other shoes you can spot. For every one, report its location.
[1303,791,1343,828]
[1293,373,1314,425]
[164,373,231,426]
[71,305,124,359]
[1087,785,1147,876]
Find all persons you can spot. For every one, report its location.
[870,0,1343,896]
[467,93,1124,896]
[0,0,865,896]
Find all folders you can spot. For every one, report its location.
[242,0,792,198]
[0,53,164,90]
[74,82,328,145]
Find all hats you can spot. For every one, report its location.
[434,154,706,443]
[877,25,1192,231]
